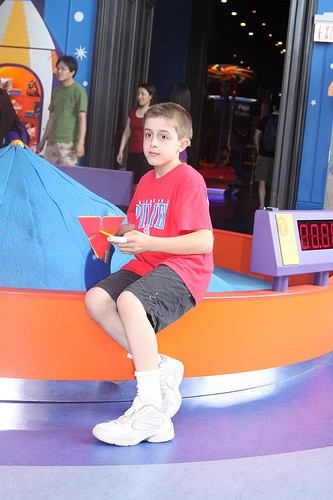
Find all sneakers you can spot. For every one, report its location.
[92,396,175,447]
[158,354,184,418]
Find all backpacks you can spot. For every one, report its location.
[259,119,278,156]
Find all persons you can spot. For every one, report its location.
[85,102,214,446]
[167,82,190,164]
[0,88,29,148]
[254,97,280,210]
[116,84,157,194]
[36,55,87,167]
